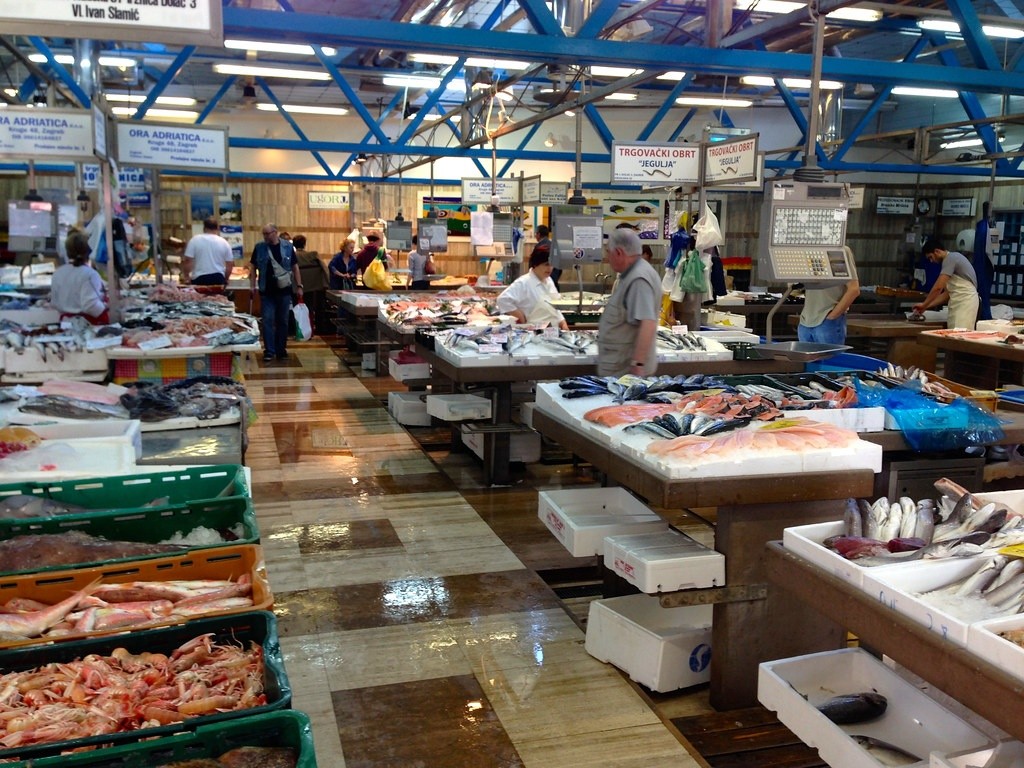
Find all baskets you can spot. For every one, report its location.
[723,341,774,361]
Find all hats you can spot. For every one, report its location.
[65,232,92,259]
[367,230,381,241]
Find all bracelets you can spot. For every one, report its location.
[630,360,645,367]
[297,285,303,288]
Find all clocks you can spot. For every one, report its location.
[918,199,932,214]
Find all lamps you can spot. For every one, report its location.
[4,0,1024,165]
[395,212,404,222]
[957,153,973,162]
[427,207,437,218]
[24,189,43,201]
[77,191,91,201]
[487,205,500,214]
[567,189,587,205]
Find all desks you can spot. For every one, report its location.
[788,314,947,371]
[531,374,1024,712]
[323,289,445,316]
[914,332,1024,386]
[374,317,418,345]
[762,540,1024,741]
[714,304,903,339]
[428,350,805,491]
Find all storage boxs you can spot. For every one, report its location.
[0,315,318,768]
[721,257,752,270]
[342,291,1024,768]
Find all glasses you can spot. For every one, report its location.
[263,231,275,236]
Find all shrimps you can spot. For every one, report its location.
[0,633,268,763]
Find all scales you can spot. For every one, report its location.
[386,220,413,273]
[899,223,924,273]
[989,229,1000,252]
[473,211,516,291]
[751,179,857,363]
[415,218,448,281]
[548,202,603,313]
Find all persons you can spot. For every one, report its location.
[797,244,860,345]
[248,223,303,360]
[183,217,234,286]
[328,238,357,335]
[355,230,388,289]
[496,249,568,330]
[597,228,662,377]
[405,235,434,290]
[279,232,329,334]
[528,225,563,291]
[386,254,395,267]
[130,241,153,274]
[642,244,653,261]
[912,239,979,369]
[50,232,109,325]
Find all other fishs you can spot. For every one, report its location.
[384,289,708,356]
[17,394,132,422]
[786,679,890,728]
[821,491,1024,615]
[847,734,925,768]
[0,525,242,571]
[559,373,857,462]
[834,361,961,398]
[0,474,237,521]
[0,574,254,641]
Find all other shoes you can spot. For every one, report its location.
[263,353,274,361]
[579,474,604,485]
[555,466,586,479]
[276,350,289,360]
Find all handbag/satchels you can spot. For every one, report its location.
[292,293,312,342]
[425,253,436,275]
[266,245,293,289]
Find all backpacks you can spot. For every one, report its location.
[362,246,393,291]
